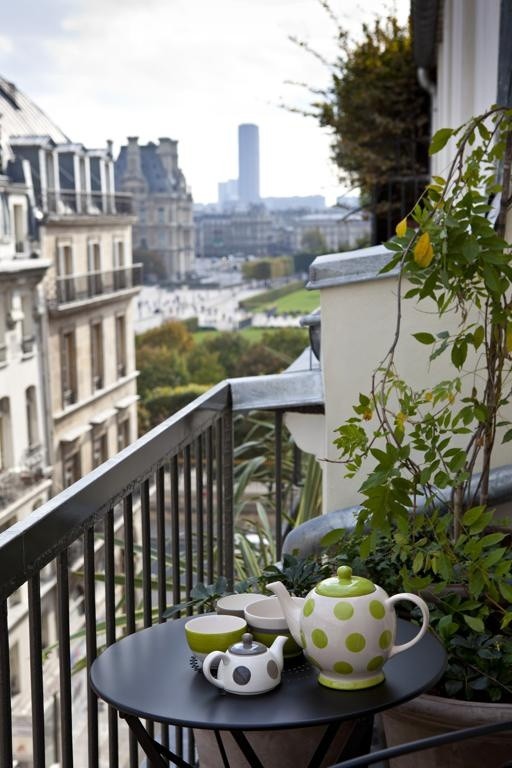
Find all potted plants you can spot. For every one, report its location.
[351,95,512,768]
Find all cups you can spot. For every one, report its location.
[215,594,269,620]
[244,595,304,659]
[184,615,247,666]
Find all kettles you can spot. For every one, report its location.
[201,629,291,698]
[265,565,430,691]
